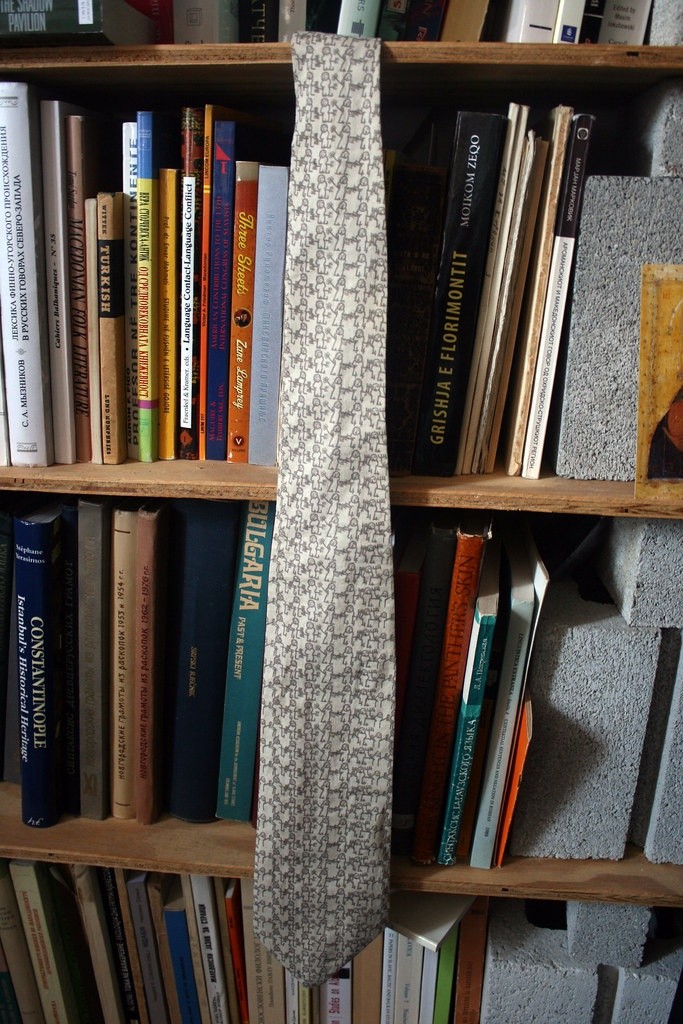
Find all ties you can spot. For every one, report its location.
[253,35,396,987]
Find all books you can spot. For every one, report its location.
[383,94,597,480]
[0,862,485,1023]
[1,0,320,50]
[389,525,547,894]
[1,500,282,831]
[377,0,678,45]
[0,79,293,472]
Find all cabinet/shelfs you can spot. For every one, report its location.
[0,0,683,1024]
